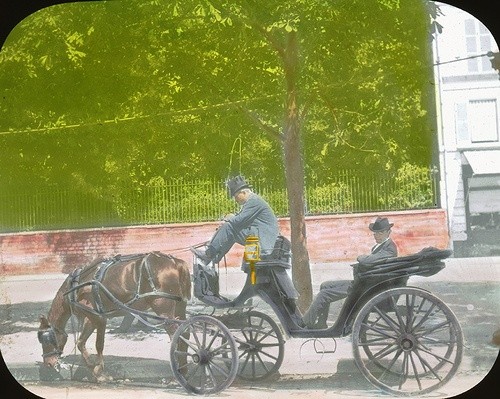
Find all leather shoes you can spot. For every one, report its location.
[307,320,328,329]
[191,248,210,265]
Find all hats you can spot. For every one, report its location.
[369,217,394,232]
[227,177,249,197]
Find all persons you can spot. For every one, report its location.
[189,176,279,265]
[295,216,397,329]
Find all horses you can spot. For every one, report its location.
[37,250,192,385]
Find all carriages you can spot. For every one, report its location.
[38,236,463,395]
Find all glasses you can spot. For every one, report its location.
[372,232,383,235]
